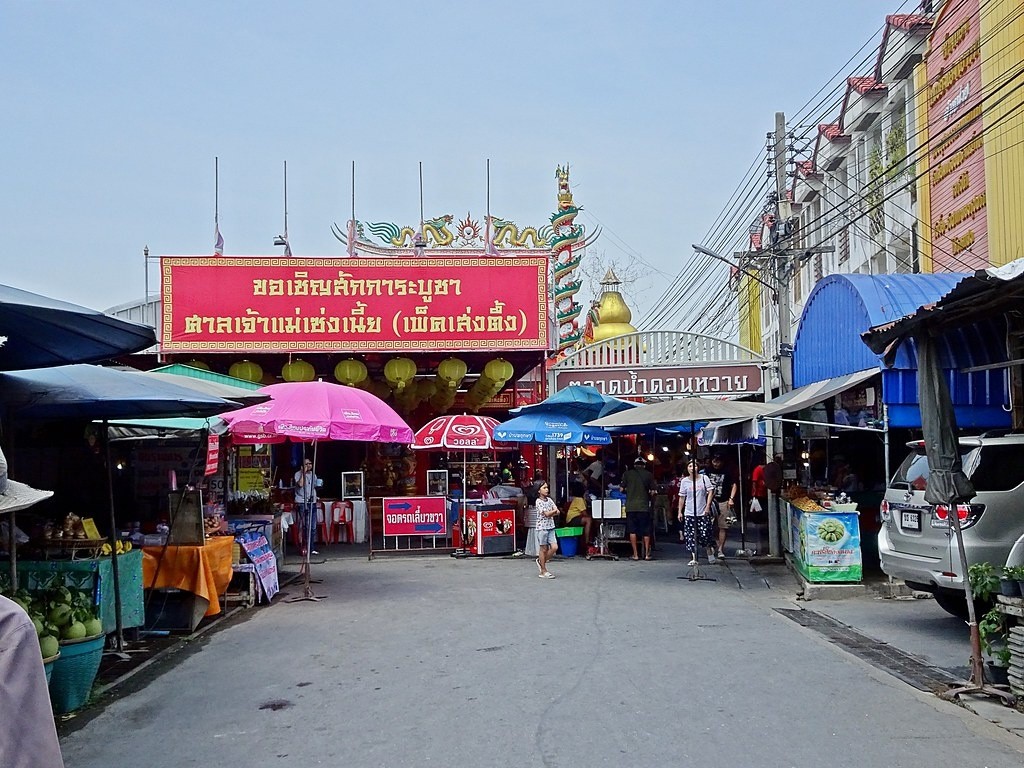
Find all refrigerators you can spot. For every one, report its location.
[453,502,516,554]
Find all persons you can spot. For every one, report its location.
[501,460,516,482]
[563,438,766,566]
[294,458,321,555]
[531,480,561,579]
[0,443,66,768]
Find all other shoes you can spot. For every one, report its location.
[718,553,725,558]
[688,560,698,566]
[311,550,319,555]
[707,547,716,564]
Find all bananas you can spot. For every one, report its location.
[101,540,133,555]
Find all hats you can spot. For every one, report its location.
[0,447,54,514]
[634,457,647,463]
[688,458,700,470]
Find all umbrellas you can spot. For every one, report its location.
[495,410,613,501]
[697,406,859,549]
[403,414,522,539]
[0,283,276,655]
[509,381,676,451]
[602,391,790,577]
[223,377,418,581]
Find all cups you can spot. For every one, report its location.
[316,479,323,486]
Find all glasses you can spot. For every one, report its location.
[305,463,312,465]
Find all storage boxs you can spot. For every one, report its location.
[600,525,624,537]
[555,527,583,537]
[592,499,622,518]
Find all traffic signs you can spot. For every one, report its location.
[383,497,447,536]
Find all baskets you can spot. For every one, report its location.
[32,537,107,549]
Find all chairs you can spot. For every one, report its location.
[281,502,354,545]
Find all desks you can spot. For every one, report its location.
[779,498,862,583]
[0,549,145,641]
[144,535,232,632]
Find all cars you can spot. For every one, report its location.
[877,432,1024,617]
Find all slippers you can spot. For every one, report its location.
[628,555,638,560]
[645,555,653,560]
[538,571,555,579]
[536,558,542,572]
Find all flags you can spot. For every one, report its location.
[214,219,502,258]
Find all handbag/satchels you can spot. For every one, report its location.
[755,499,762,512]
[703,474,721,517]
[726,509,737,527]
[748,496,755,513]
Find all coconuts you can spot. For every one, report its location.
[0,586,102,657]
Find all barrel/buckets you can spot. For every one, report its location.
[557,536,577,557]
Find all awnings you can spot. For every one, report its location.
[703,362,887,435]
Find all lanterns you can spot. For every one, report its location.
[334,355,367,387]
[384,355,418,388]
[185,358,210,371]
[228,358,264,383]
[280,358,316,382]
[485,358,515,387]
[437,357,467,388]
[260,369,507,414]
